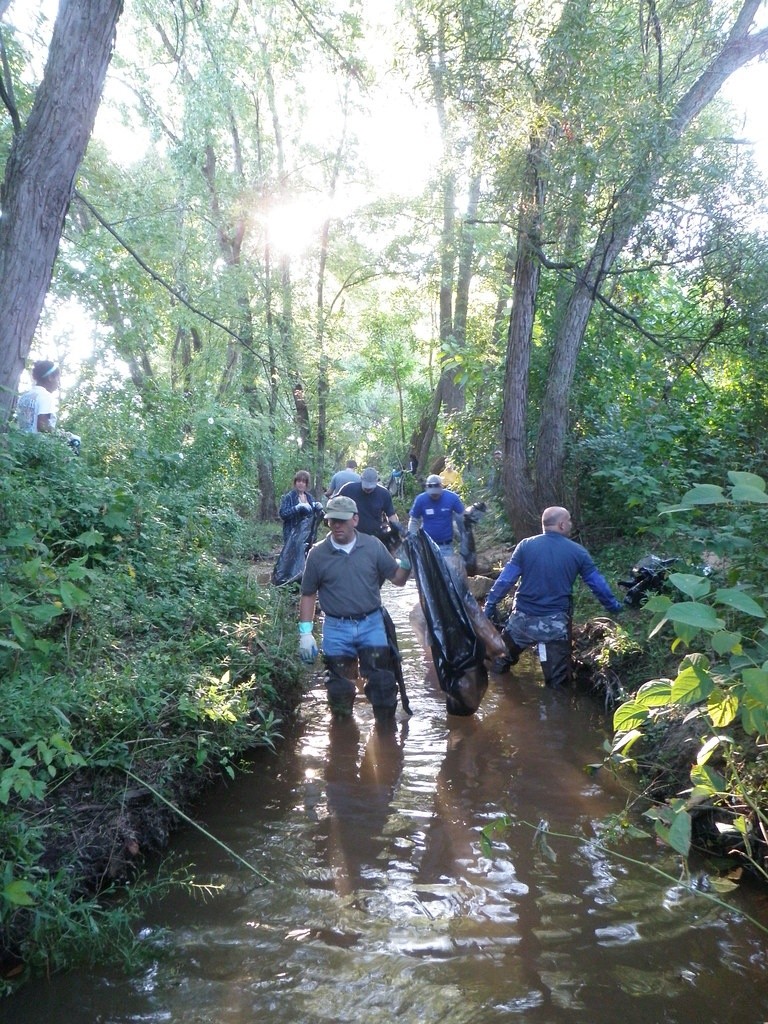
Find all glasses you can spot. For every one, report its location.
[426,483,442,488]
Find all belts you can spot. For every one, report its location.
[436,539,452,545]
[325,607,380,620]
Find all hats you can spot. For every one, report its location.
[426,474,443,496]
[360,467,378,489]
[324,495,358,520]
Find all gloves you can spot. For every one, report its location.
[297,622,318,665]
[398,546,412,569]
[484,562,521,619]
[582,569,626,613]
[68,433,81,447]
[311,501,323,511]
[295,502,311,513]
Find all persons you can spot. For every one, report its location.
[17,360,81,448]
[484,506,624,691]
[406,454,418,476]
[332,468,404,589]
[323,459,361,499]
[438,457,463,493]
[391,466,405,478]
[297,496,413,721]
[279,470,323,558]
[408,474,487,553]
